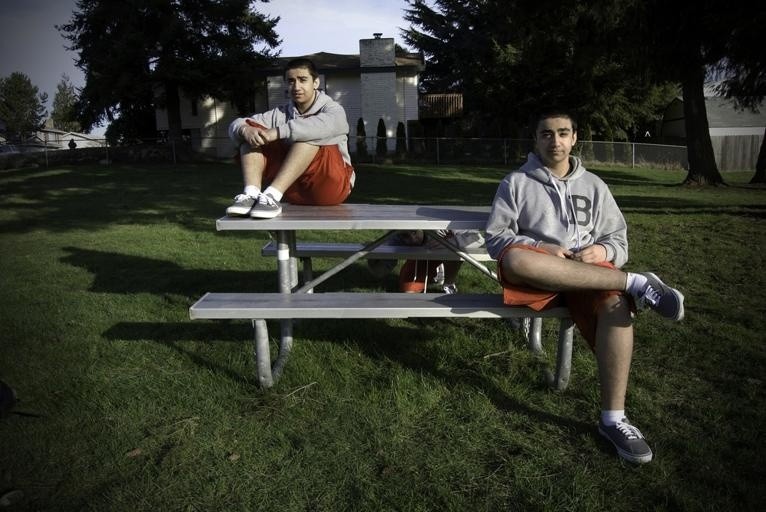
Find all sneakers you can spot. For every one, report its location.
[442,282,459,294]
[227,192,283,219]
[599,416,653,464]
[640,271,686,324]
[434,264,445,286]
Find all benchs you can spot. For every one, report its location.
[190,292,576,390]
[261,244,497,293]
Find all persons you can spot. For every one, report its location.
[360,231,486,294]
[225,57,357,220]
[485,102,686,463]
[69,138,76,149]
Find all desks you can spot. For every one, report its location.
[216,203,573,393]
